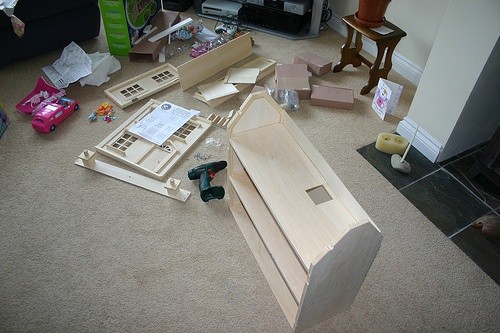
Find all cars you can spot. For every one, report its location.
[31,97,80,135]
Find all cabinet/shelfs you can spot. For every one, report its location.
[227,91,384,333]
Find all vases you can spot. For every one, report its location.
[358,0,391,22]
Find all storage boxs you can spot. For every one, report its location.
[311,85,354,111]
[294,51,333,76]
[98,0,181,63]
[275,64,311,100]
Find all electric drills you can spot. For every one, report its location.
[188,160,227,203]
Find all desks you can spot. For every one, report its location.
[332,14,407,95]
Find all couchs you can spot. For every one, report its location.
[0,0,100,66]
